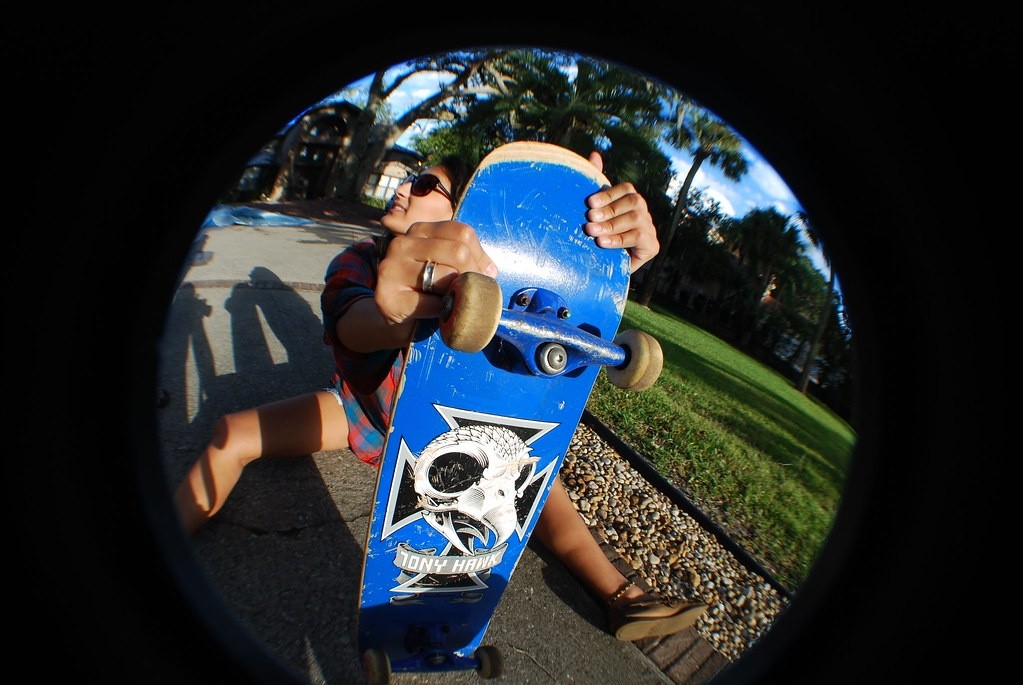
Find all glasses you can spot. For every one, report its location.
[402,174,457,203]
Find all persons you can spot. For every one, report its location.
[175,151,712,643]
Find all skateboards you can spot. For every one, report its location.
[352,142,632,684]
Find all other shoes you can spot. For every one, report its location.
[612,594,709,641]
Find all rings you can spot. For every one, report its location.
[422,261,436,293]
